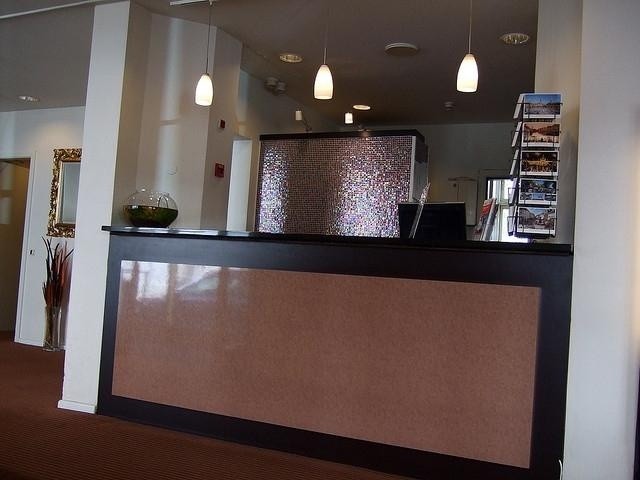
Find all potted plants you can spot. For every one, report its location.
[37,235,75,352]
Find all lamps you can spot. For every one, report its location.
[456,1,480,95]
[312,2,335,101]
[194,2,216,106]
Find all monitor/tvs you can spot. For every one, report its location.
[398,203,466,240]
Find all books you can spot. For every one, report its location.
[508,94,524,232]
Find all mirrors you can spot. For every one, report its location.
[46,147,83,239]
